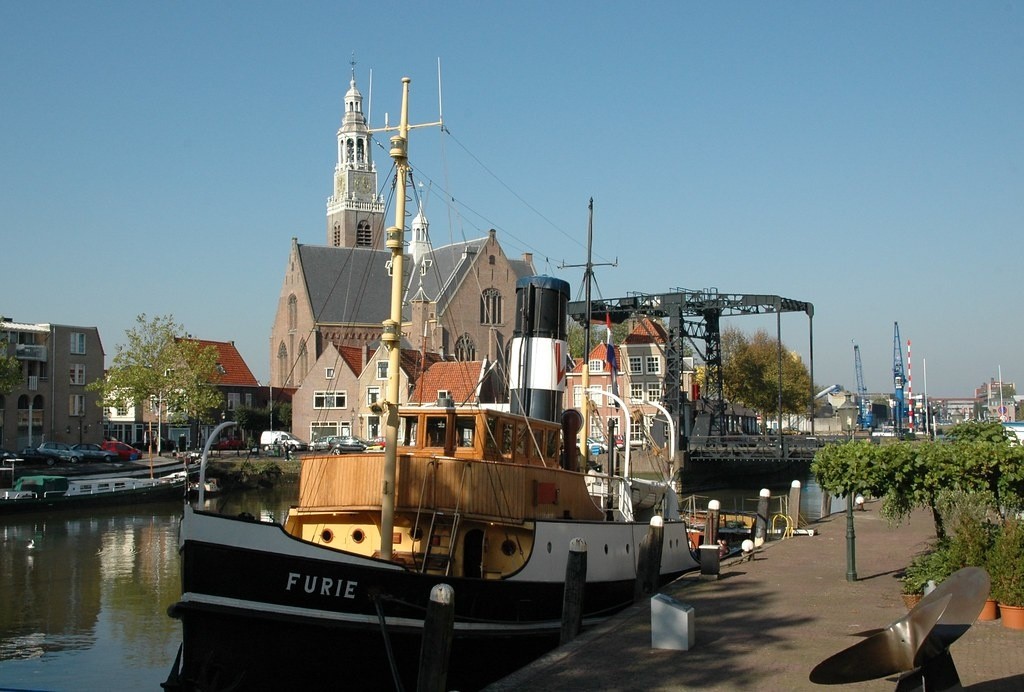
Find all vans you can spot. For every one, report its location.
[328,435,370,456]
[260,430,308,452]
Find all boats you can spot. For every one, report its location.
[160,60,754,692]
[0,465,188,511]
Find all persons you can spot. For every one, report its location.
[283,440,289,461]
[273,438,278,447]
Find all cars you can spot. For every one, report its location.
[102,441,143,462]
[214,435,247,451]
[0,450,18,464]
[366,436,386,447]
[130,437,177,453]
[71,443,120,464]
[37,442,85,465]
[310,436,328,452]
[16,446,63,467]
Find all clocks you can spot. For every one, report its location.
[336,176,346,196]
[355,176,372,193]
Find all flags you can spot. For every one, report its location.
[605,311,620,412]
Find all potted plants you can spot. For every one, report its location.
[896,491,1024,629]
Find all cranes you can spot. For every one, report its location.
[892,321,919,443]
[851,338,876,437]
